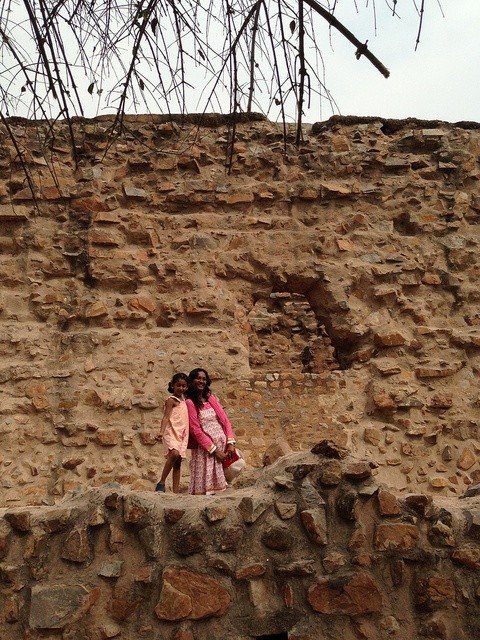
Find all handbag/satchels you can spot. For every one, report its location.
[222,448,246,482]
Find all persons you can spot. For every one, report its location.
[185,369,235,495]
[155,373,189,494]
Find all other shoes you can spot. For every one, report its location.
[156,483,165,493]
